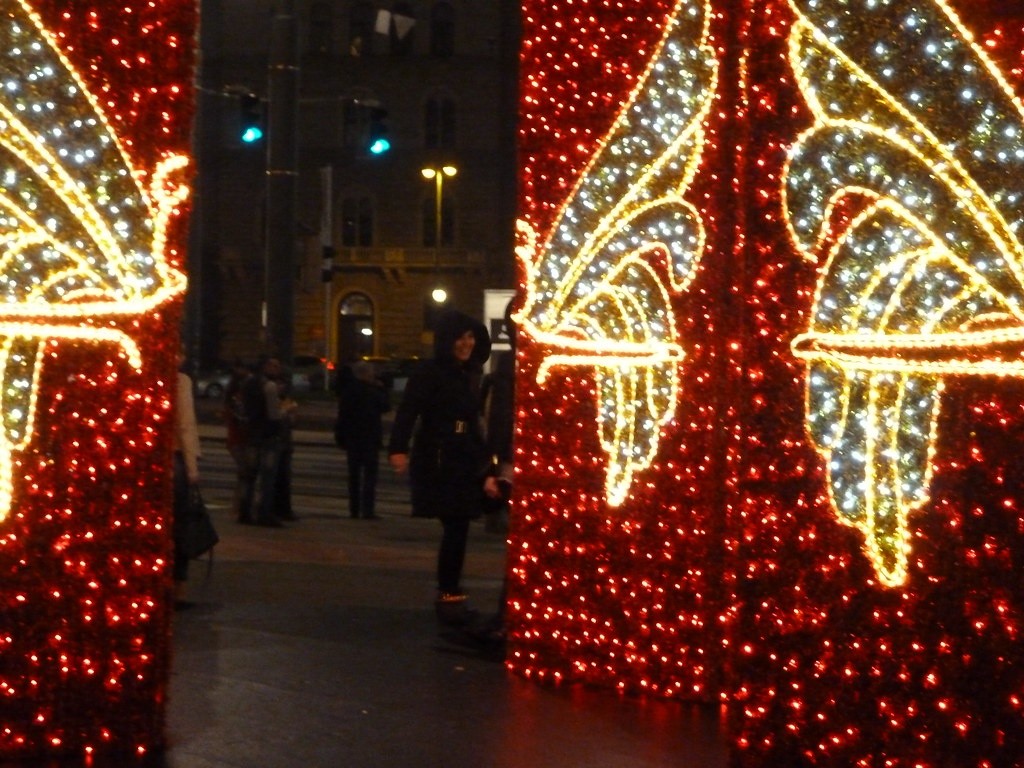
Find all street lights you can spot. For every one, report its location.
[421,165,459,335]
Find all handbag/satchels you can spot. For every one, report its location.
[172,485,220,564]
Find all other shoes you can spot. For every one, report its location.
[236,508,259,526]
[434,599,480,626]
[273,509,300,521]
[349,500,379,519]
[256,505,284,528]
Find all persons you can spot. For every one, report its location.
[175,340,514,608]
[386,313,501,608]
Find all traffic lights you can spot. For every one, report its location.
[240,94,264,147]
[369,109,391,159]
